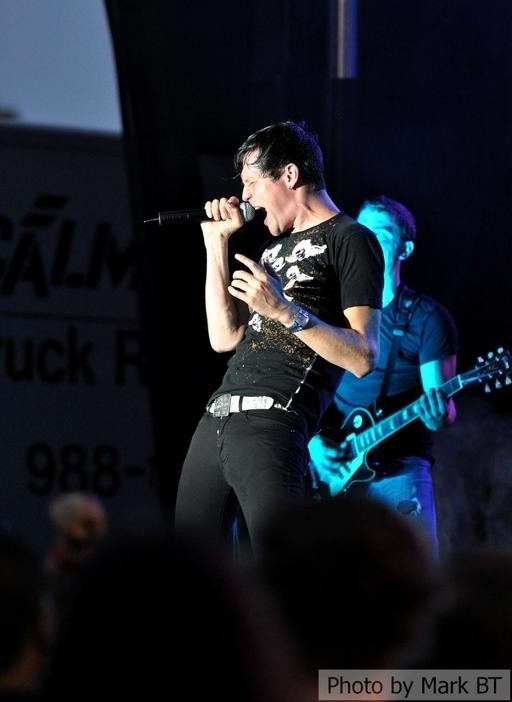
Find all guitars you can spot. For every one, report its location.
[306,348,512,500]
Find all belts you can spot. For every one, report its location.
[205,387,302,419]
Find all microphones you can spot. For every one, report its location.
[142,203,257,224]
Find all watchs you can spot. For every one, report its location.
[285,308,309,334]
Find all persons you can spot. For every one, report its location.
[307,197,459,563]
[170,120,385,558]
